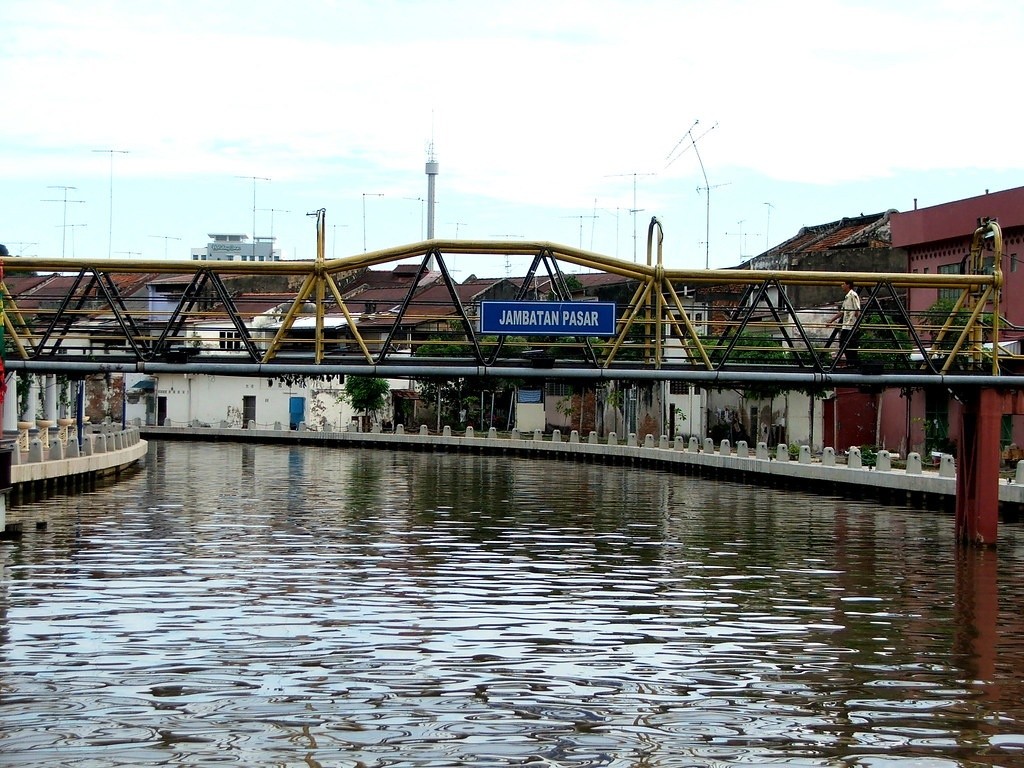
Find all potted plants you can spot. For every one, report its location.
[768,447,776,461]
[788,444,799,461]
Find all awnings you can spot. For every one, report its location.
[125,381,156,395]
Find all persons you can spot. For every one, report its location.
[825,281,864,367]
